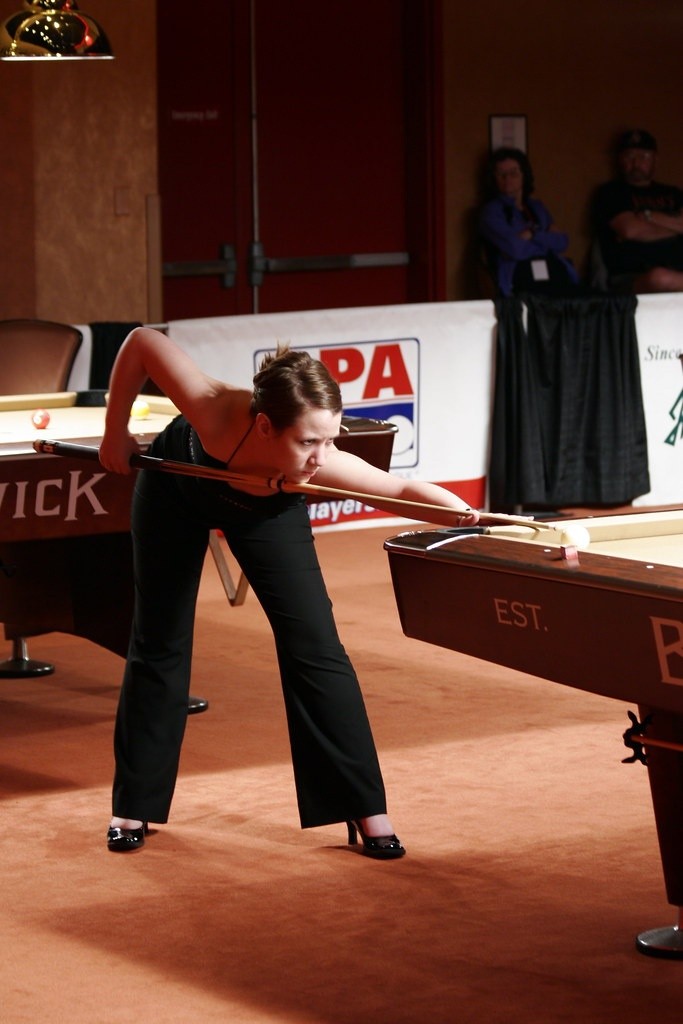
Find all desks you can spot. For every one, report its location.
[0,389,250,716]
[384,502,683,959]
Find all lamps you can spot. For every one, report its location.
[0,0,117,61]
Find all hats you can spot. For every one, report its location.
[615,129,655,153]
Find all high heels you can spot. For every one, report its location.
[107,816,149,849]
[346,819,406,857]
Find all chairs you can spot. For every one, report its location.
[0,318,83,396]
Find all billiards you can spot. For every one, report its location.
[31,409,50,429]
[560,525,591,550]
[130,400,150,420]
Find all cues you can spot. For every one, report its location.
[34,438,559,534]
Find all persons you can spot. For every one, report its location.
[476,147,581,296]
[95,328,535,862]
[583,130,683,293]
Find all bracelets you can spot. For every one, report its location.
[645,209,652,221]
